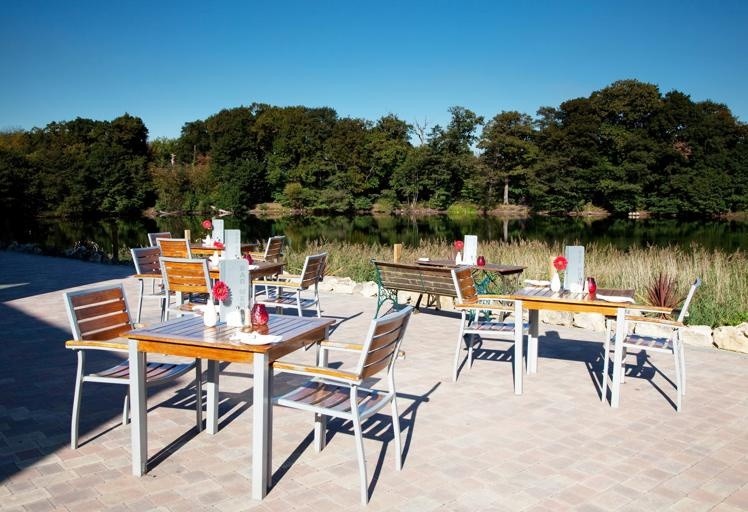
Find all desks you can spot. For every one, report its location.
[510,284,635,408]
[128,308,338,499]
[414,258,527,322]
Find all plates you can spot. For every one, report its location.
[524,278,549,286]
[596,293,636,304]
[231,331,282,346]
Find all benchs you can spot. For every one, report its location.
[371,258,490,327]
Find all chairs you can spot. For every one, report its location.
[63,283,203,474]
[450,266,532,382]
[268,305,415,503]
[130,233,328,357]
[601,277,701,411]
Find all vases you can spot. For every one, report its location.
[203,299,217,329]
[251,303,268,326]
[206,235,211,246]
[213,251,219,266]
[243,253,254,265]
[456,252,461,265]
[551,271,561,292]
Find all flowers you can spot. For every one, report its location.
[213,241,225,252]
[202,221,212,235]
[454,241,464,252]
[210,281,229,302]
[553,256,568,271]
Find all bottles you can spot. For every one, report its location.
[477,255,485,266]
[203,299,217,327]
[587,277,596,294]
[227,304,269,327]
